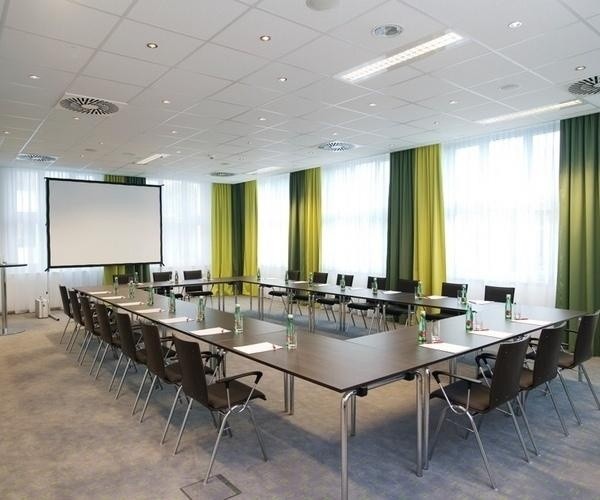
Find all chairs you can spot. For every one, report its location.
[138,321,219,445]
[479,320,570,457]
[429,336,531,492]
[174,335,268,485]
[58,268,515,423]
[526,312,600,426]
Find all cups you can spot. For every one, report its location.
[234,314,244,332]
[427,321,441,342]
[287,327,297,350]
[472,313,483,331]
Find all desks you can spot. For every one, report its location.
[346,305,587,468]
[217,329,424,498]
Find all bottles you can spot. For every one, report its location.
[417,313,426,343]
[234,304,241,320]
[113,271,211,322]
[285,314,293,346]
[256,267,377,295]
[415,281,521,320]
[465,302,473,330]
